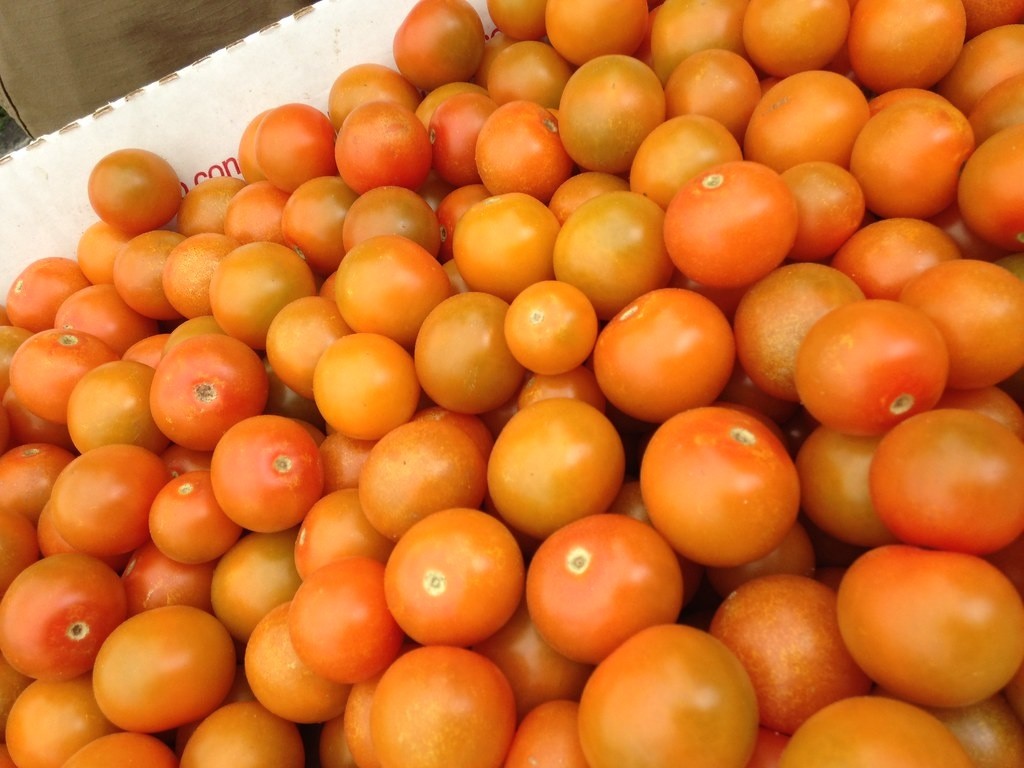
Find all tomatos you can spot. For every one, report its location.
[1,0,1024,767]
